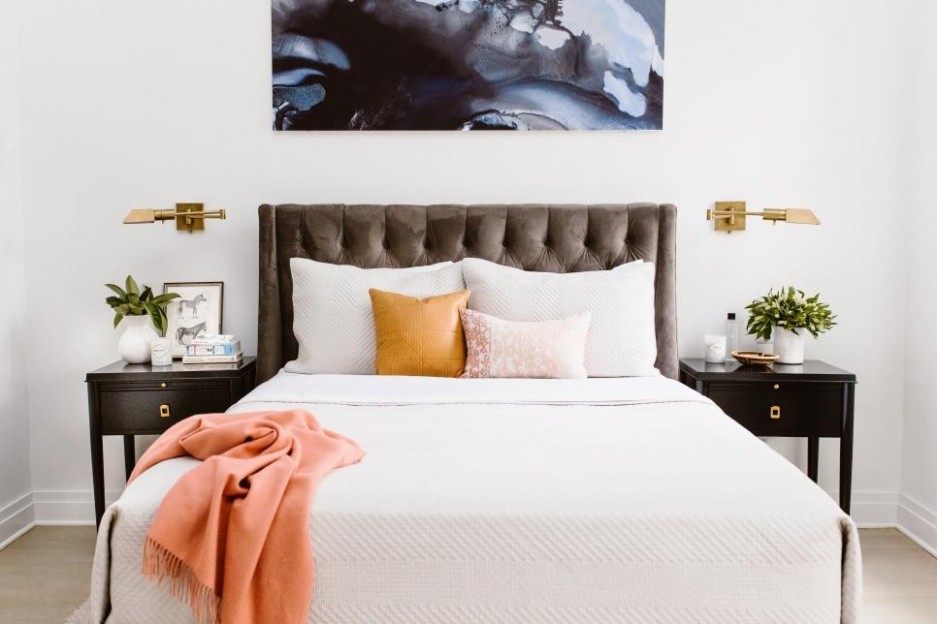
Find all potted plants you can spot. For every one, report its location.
[103,274,181,365]
[743,286,840,366]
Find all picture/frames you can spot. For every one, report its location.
[162,281,224,361]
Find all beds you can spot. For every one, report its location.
[66,205,863,623]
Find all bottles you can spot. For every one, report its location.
[725,313,738,364]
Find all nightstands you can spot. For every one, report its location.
[84,356,257,534]
[678,358,859,516]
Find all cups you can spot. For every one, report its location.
[706,333,727,364]
[150,336,173,366]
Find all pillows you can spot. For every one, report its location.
[285,256,467,375]
[461,257,661,377]
[458,307,591,380]
[369,289,472,377]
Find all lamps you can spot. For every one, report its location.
[706,200,821,232]
[122,203,226,233]
